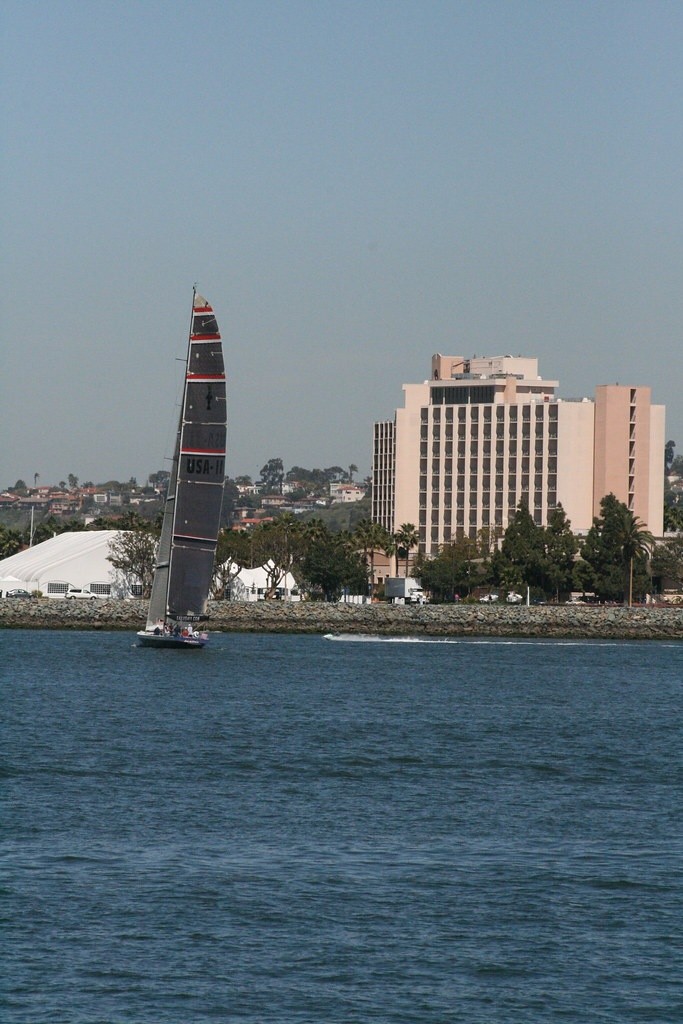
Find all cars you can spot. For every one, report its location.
[531,598,546,605]
[6,588,33,599]
[65,588,99,600]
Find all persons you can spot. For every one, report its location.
[418,594,431,606]
[454,592,513,605]
[154,622,199,638]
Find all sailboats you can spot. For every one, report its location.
[135,286,229,650]
